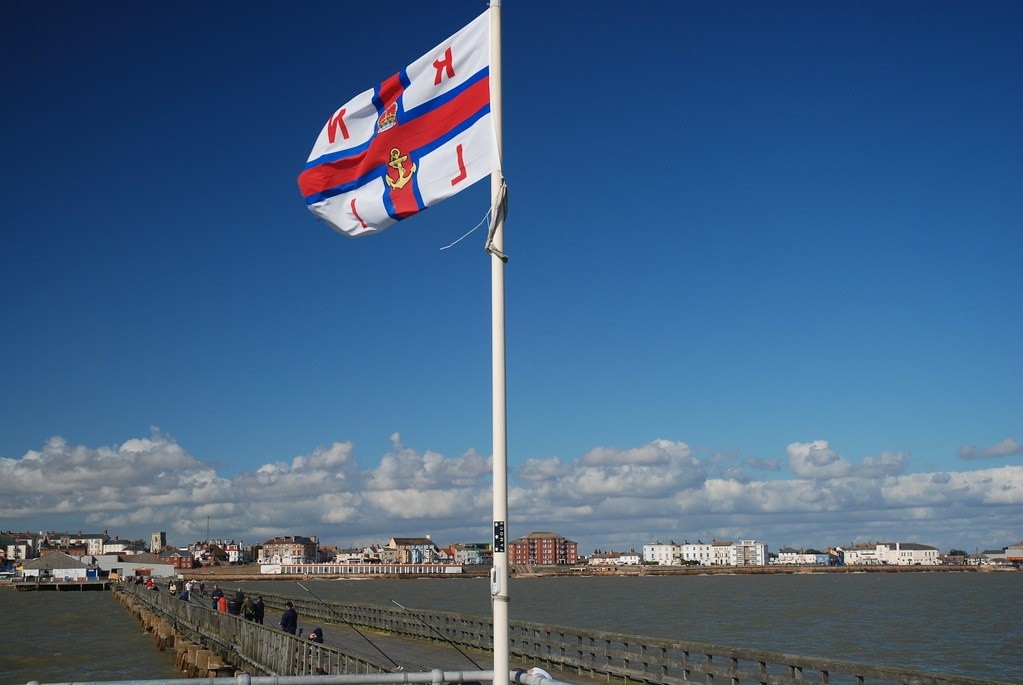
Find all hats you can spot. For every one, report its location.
[285,602,293,607]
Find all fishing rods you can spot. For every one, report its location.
[389,598,485,671]
[263,616,278,630]
[296,581,404,670]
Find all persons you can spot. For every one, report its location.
[125,575,159,591]
[168,579,193,601]
[119,574,122,580]
[279,602,298,635]
[228,589,265,625]
[211,585,226,612]
[308,628,323,670]
[199,580,208,598]
[512,667,552,685]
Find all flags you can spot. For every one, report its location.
[298,0,502,238]
